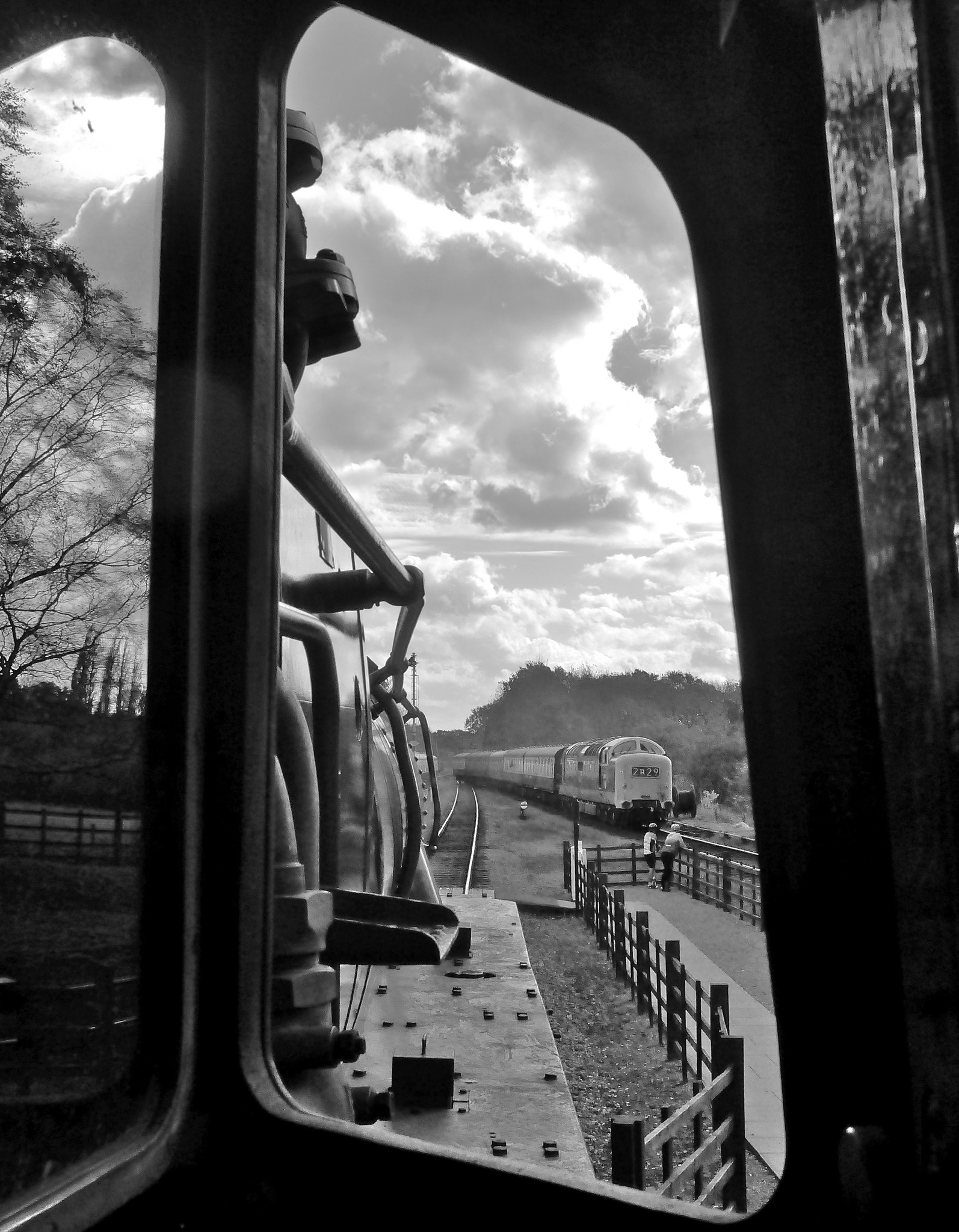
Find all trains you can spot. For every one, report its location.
[449,736,674,826]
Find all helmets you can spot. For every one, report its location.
[671,823,680,831]
[648,823,657,828]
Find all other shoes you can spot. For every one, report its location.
[652,878,656,888]
[662,887,672,892]
[647,884,659,889]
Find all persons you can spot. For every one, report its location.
[661,824,685,892]
[643,822,660,889]
